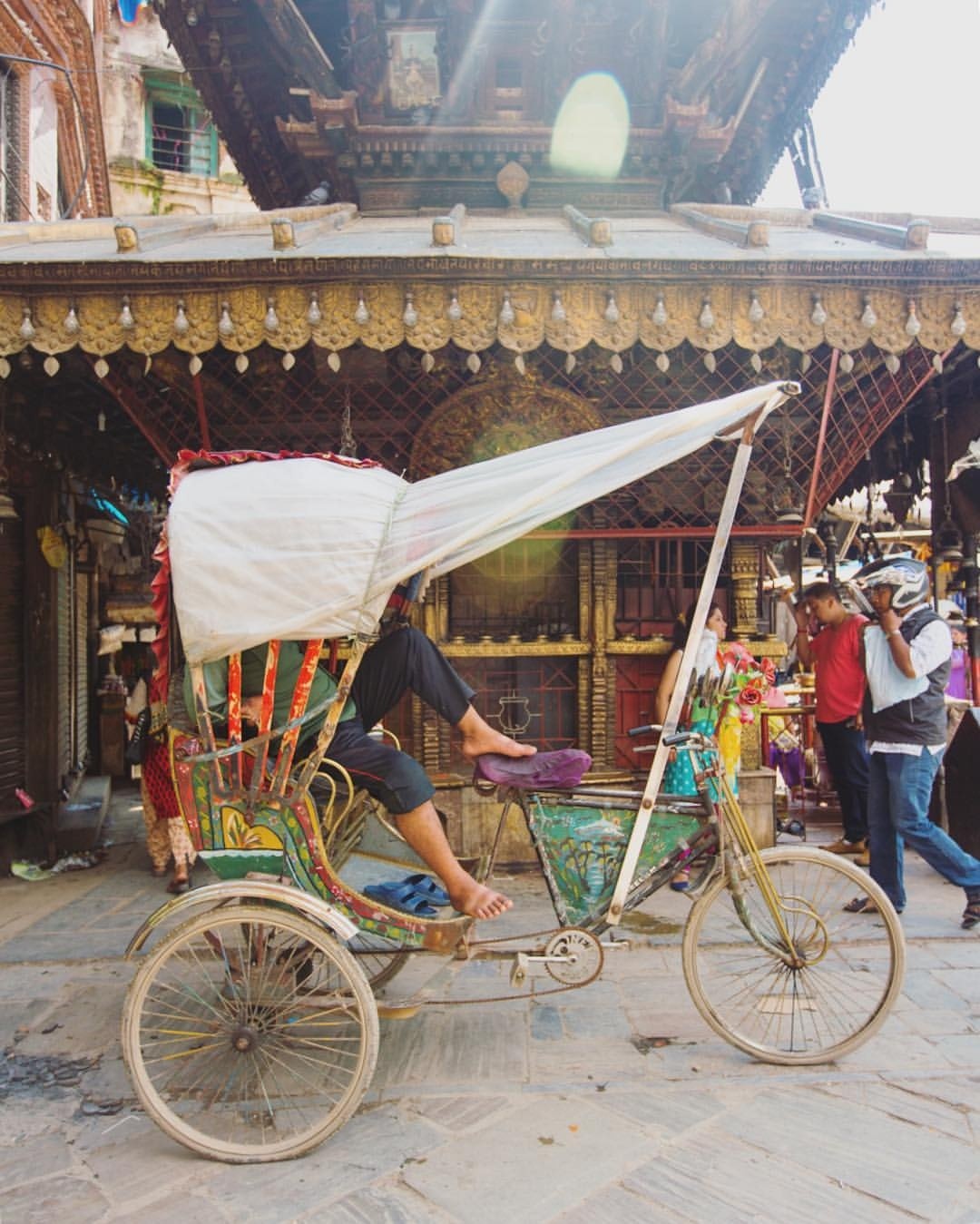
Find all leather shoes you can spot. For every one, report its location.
[818,837,866,854]
[854,850,871,866]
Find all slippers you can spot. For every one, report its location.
[364,884,438,918]
[385,874,451,906]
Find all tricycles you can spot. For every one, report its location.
[114,380,908,1172]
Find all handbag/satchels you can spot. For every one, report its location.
[123,667,152,768]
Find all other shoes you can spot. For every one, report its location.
[961,899,980,928]
[153,865,166,876]
[843,896,904,914]
[670,879,688,892]
[167,878,189,893]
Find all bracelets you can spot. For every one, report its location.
[885,629,901,639]
[798,629,807,633]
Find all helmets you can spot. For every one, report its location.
[864,558,931,610]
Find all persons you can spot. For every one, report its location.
[182,625,540,924]
[125,661,199,893]
[844,556,980,929]
[949,624,973,698]
[656,598,744,891]
[792,579,873,868]
